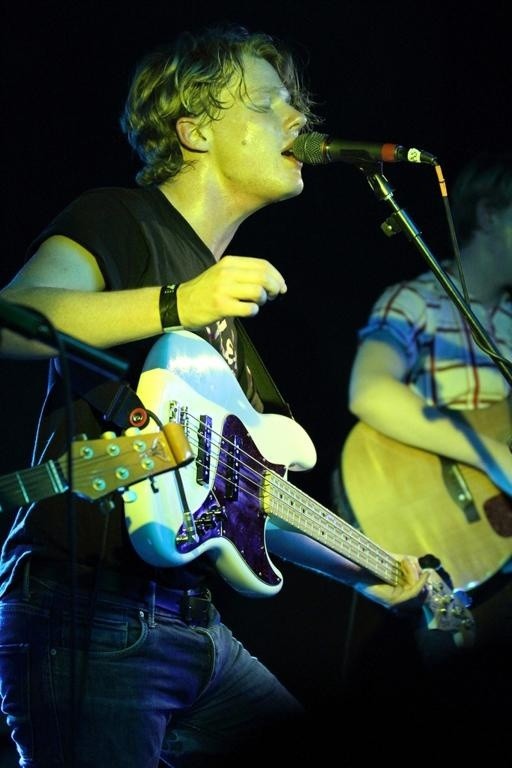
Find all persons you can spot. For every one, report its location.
[345,152,511,767]
[0,27,430,768]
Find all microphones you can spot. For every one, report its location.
[291,131,437,165]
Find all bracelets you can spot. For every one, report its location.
[160,283,182,332]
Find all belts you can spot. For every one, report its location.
[14,557,221,628]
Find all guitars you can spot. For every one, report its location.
[111,330,474,634]
[334,398,511,595]
